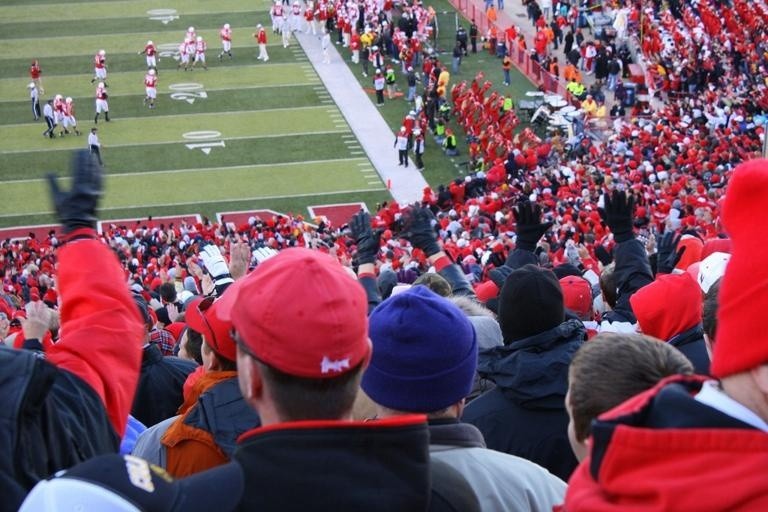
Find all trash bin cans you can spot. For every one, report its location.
[620,81,637,109]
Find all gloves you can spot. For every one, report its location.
[48,149,101,231]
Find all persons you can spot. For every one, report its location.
[1,1,768,507]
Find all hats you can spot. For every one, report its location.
[18,454,244,511]
[698,158,768,377]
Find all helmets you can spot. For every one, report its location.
[27,23,262,133]
